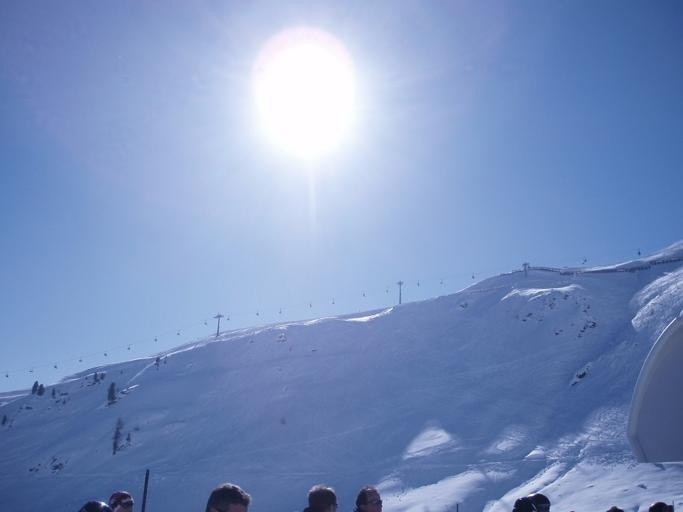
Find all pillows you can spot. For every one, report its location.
[513,493,550,512]
[108,491,133,511]
[78,501,113,512]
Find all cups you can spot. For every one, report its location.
[119,501,133,508]
[367,499,382,504]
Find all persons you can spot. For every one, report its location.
[351,485,382,511]
[205,483,252,511]
[107,490,134,512]
[301,484,337,511]
